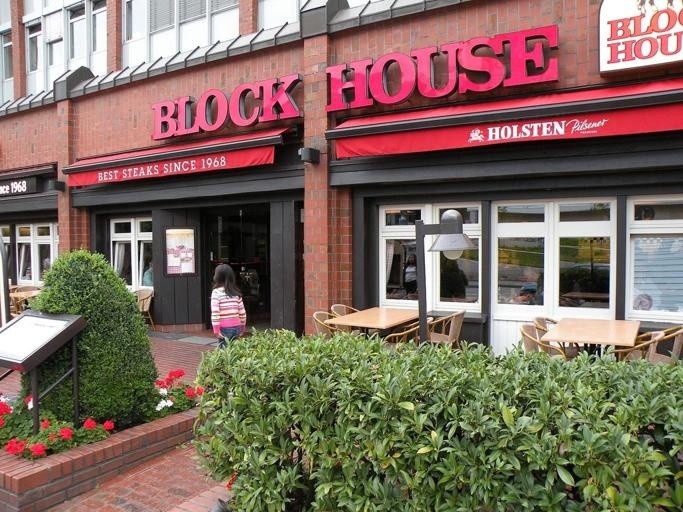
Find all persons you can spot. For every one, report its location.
[211,264,247,350]
[143,262,153,286]
[403,254,417,295]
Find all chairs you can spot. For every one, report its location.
[517,314,682,365]
[6,281,156,333]
[309,301,467,355]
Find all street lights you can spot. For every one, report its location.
[415,209,471,347]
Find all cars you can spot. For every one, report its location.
[514,259,609,306]
